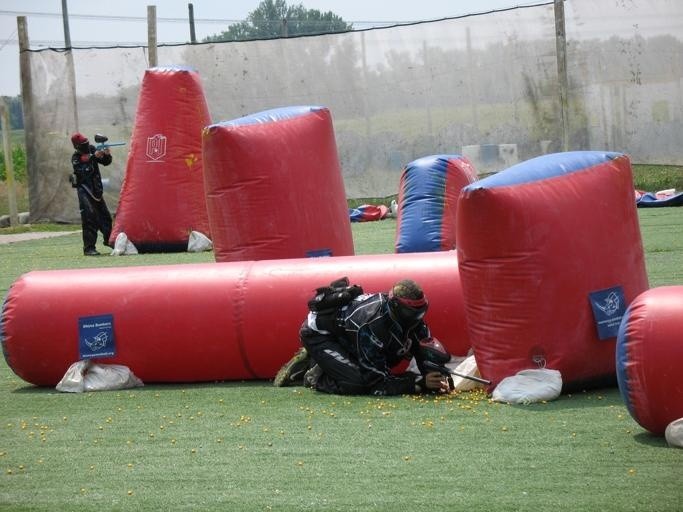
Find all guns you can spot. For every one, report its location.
[419,337,491,394]
[94,134,126,159]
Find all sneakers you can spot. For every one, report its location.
[83,246,101,256]
[273,347,316,388]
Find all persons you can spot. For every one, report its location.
[70,132,112,256]
[273,278,451,396]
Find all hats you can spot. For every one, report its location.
[71,132,87,147]
[388,280,428,314]
